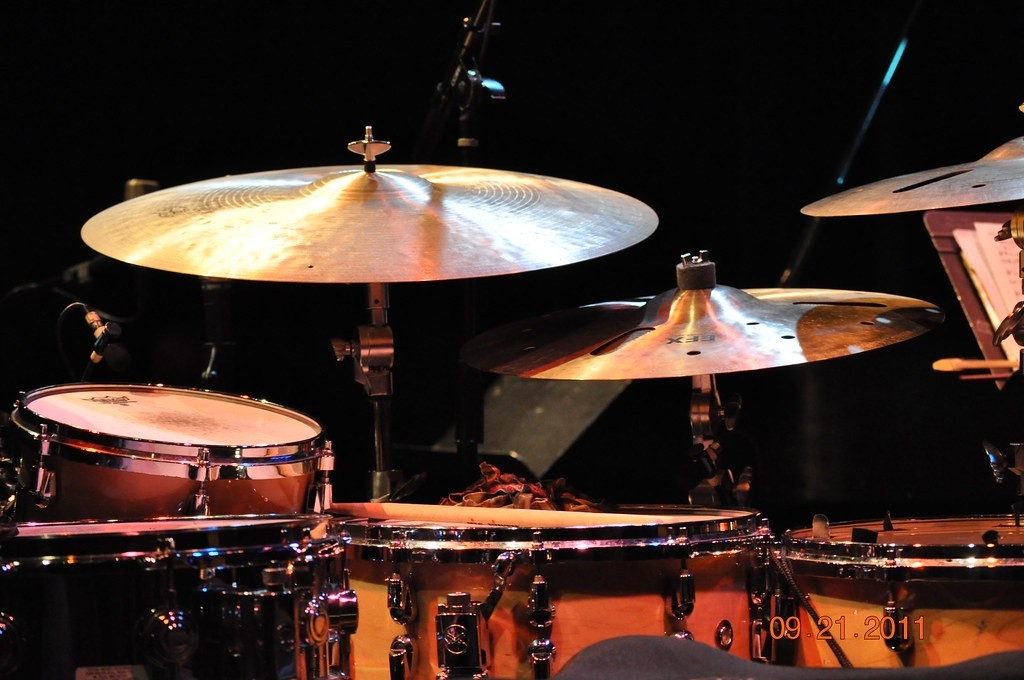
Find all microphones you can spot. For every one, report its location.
[81,322,123,382]
[409,0,496,164]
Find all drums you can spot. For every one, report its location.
[287,497,787,680]
[750,512,1023,674]
[1,508,364,680]
[5,380,338,576]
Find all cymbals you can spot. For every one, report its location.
[449,285,951,384]
[798,135,1024,220]
[79,160,663,287]
